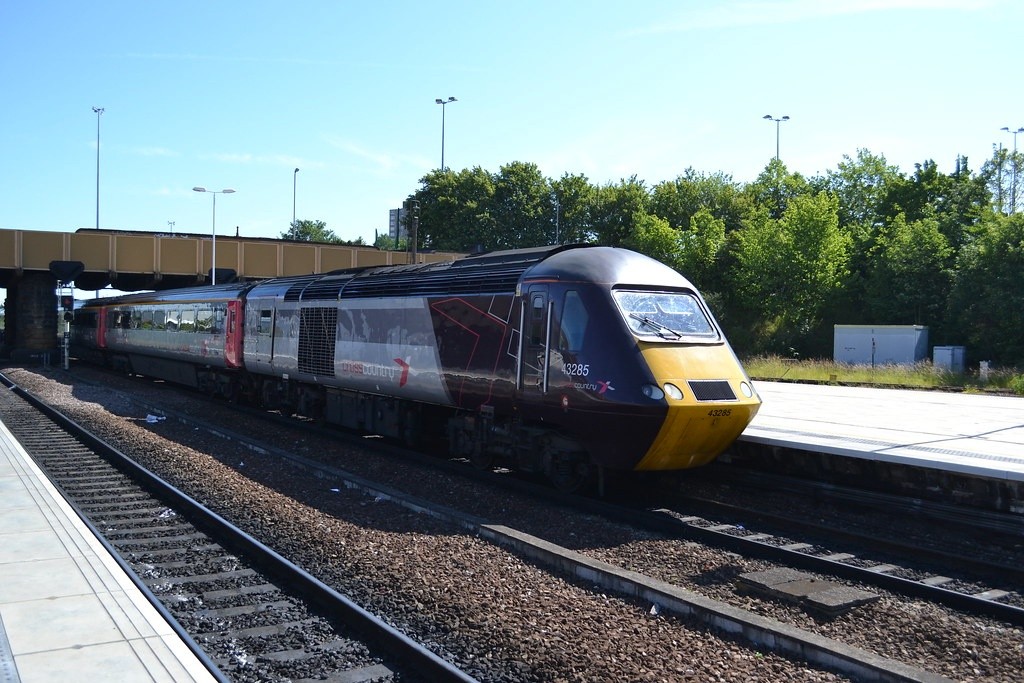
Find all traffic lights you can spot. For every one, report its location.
[61,297,74,309]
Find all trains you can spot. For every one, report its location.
[71,243,764,482]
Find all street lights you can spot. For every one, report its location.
[193,186,236,285]
[293,168,300,244]
[91,106,105,233]
[434,96,457,176]
[999,125,1024,218]
[763,114,789,209]
